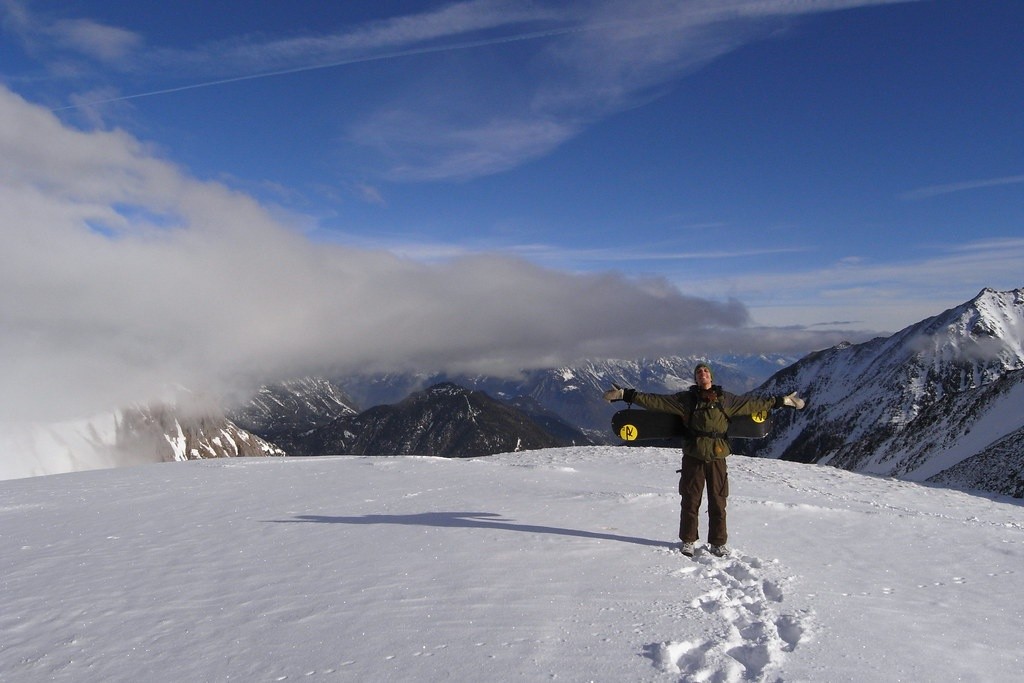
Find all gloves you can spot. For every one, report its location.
[782,391,805,410]
[602,382,624,401]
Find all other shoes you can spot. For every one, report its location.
[680,540,695,556]
[711,544,731,556]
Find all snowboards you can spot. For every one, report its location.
[610,409,773,442]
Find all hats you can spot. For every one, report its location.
[694,363,713,379]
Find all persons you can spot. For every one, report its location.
[601,363,805,557]
